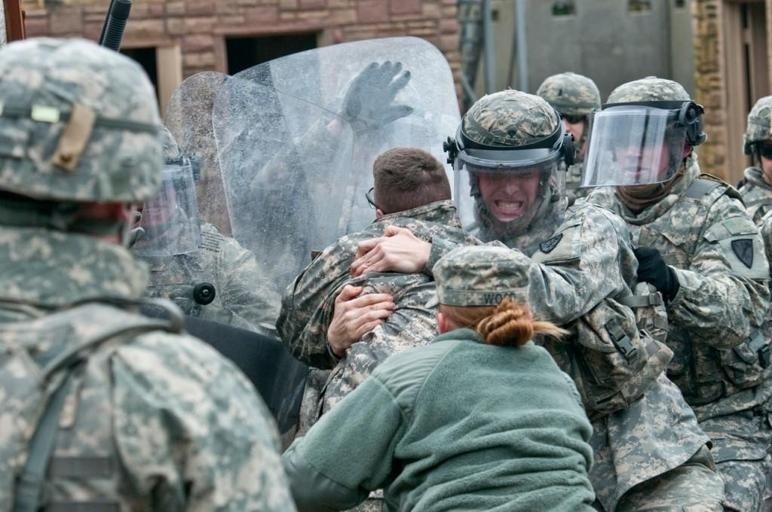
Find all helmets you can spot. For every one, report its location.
[443,90,576,259]
[0,36,164,204]
[578,76,706,188]
[742,95,772,154]
[127,124,202,259]
[536,73,602,145]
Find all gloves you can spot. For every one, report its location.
[633,246,680,302]
[339,60,415,134]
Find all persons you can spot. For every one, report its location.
[132,123,281,341]
[537,72,604,196]
[735,96,772,268]
[281,243,598,512]
[577,75,772,512]
[1,36,300,512]
[323,89,727,512]
[244,61,414,290]
[276,147,481,512]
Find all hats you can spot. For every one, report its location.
[431,245,530,307]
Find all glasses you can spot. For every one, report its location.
[365,187,387,215]
[559,113,585,124]
[755,142,772,160]
